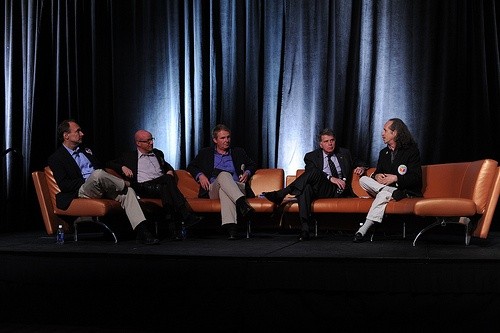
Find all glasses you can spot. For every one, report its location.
[139,138,155,143]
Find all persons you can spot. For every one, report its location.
[111,129,202,243]
[48,120,159,244]
[262,128,372,240]
[186,124,257,240]
[352,118,422,243]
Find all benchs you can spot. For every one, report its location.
[31,159,500,243]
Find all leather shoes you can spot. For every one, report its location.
[227,226,240,240]
[262,190,283,205]
[353,233,363,242]
[175,231,186,239]
[240,203,254,215]
[297,232,309,241]
[393,188,415,200]
[136,231,160,245]
[182,215,203,227]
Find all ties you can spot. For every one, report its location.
[328,154,339,179]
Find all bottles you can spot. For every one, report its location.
[337,178,346,194]
[180,221,186,239]
[57,225,64,244]
